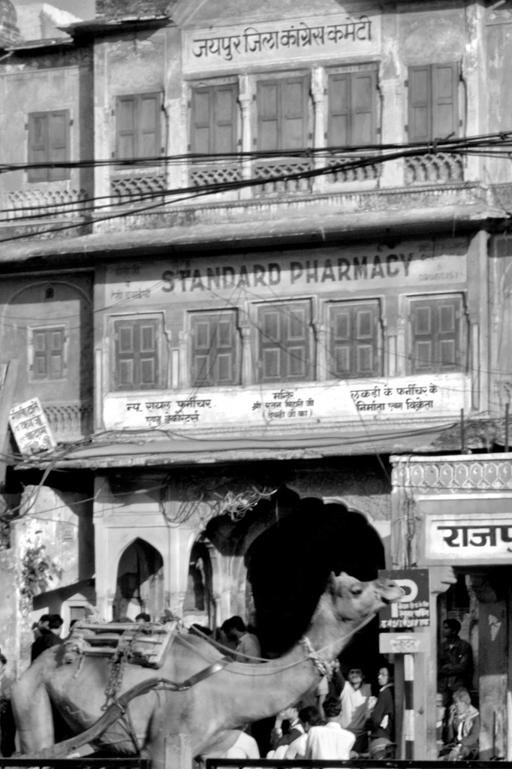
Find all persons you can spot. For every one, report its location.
[0,648,16,758]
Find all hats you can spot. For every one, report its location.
[369,738,397,760]
[342,660,375,683]
